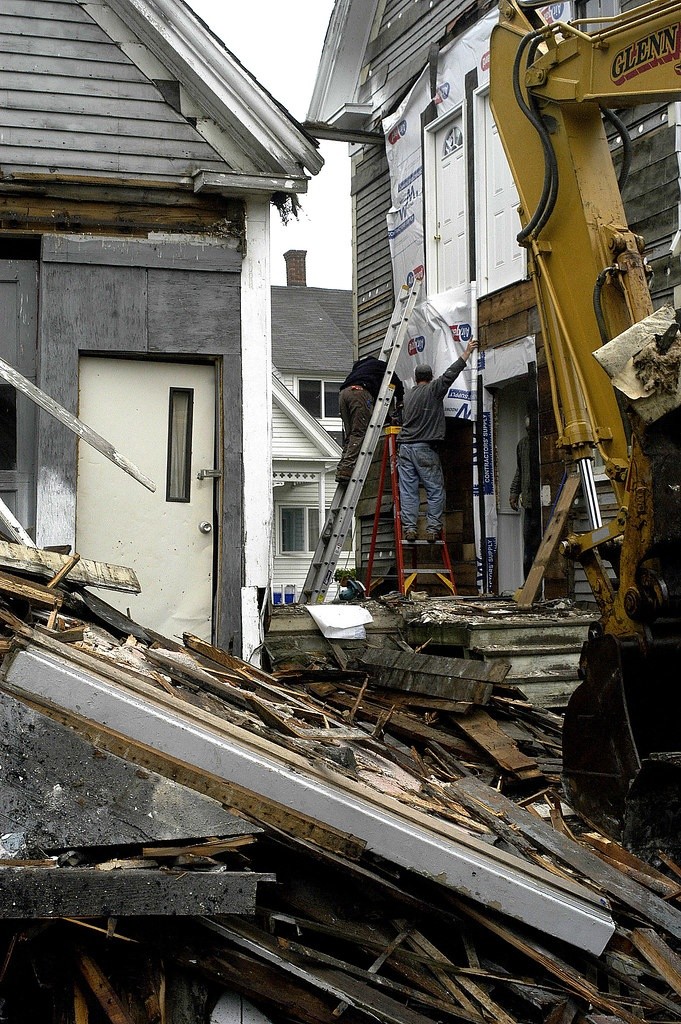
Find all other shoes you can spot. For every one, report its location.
[425,533,439,541]
[406,535,416,540]
[335,470,353,482]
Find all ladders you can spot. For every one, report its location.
[297,279,422,605]
[365,426,458,600]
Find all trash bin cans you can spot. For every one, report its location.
[273,583,297,605]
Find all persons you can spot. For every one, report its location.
[509,416,541,580]
[396,335,480,542]
[335,356,406,482]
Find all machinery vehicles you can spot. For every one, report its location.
[488,0,680,847]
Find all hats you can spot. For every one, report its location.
[415,363,432,373]
[357,355,378,361]
